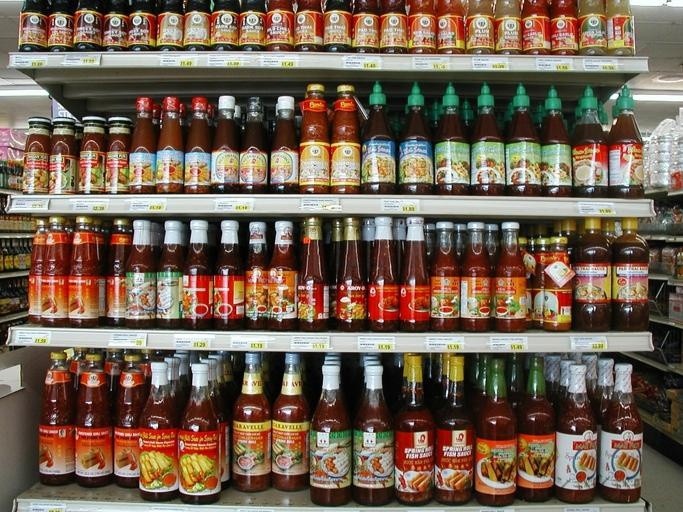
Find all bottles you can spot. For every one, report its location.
[19,0,638,56]
[22,79,643,198]
[29,215,650,332]
[676,247,683,280]
[36,351,645,507]
[0,146,35,354]
[641,108,683,193]
